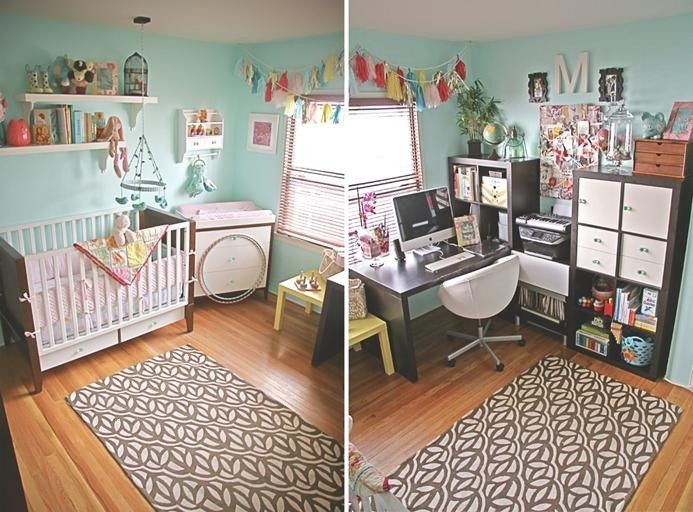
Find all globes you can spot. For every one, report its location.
[482,122,507,160]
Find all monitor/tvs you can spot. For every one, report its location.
[393,186,456,256]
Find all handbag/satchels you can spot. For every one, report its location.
[319,250,344,282]
[349,278,368,320]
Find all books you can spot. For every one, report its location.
[34,104,107,144]
[452,162,476,203]
[577,334,605,353]
[615,283,641,326]
[581,322,612,338]
[520,285,565,322]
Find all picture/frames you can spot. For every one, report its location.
[246,113,278,154]
[528,72,548,103]
[453,214,479,246]
[598,68,624,102]
[663,101,692,141]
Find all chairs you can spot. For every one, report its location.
[437,254,526,371]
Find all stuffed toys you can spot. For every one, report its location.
[0,93,9,148]
[24,54,94,93]
[115,181,168,210]
[185,152,217,198]
[185,108,221,136]
[577,276,614,316]
[97,116,130,178]
[112,211,137,245]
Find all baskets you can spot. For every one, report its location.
[592,273,614,300]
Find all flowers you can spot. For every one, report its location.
[357,191,377,229]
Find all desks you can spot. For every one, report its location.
[348,241,509,383]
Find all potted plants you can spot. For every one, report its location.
[455,77,502,157]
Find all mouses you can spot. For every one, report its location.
[492,238,502,244]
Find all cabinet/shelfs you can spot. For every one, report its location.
[447,156,539,327]
[177,108,223,163]
[633,139,693,179]
[512,247,569,350]
[174,200,275,303]
[568,167,693,382]
[0,93,157,155]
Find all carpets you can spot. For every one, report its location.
[65,343,343,511]
[386,352,684,511]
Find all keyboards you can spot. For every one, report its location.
[423,252,476,274]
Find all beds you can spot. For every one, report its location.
[0,204,195,394]
[273,270,326,331]
